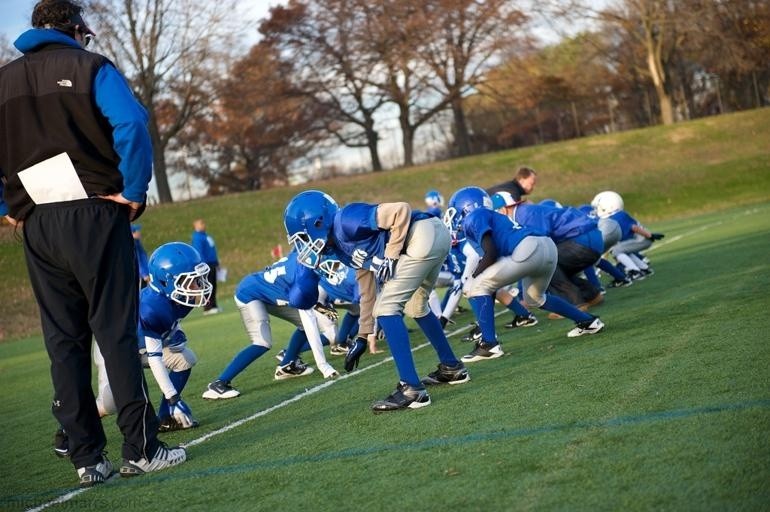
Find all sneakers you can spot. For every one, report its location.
[202,382,240,400]
[274,338,358,380]
[566,318,605,337]
[54,428,69,458]
[76,450,113,487]
[460,327,497,342]
[421,362,471,385]
[504,316,538,329]
[599,255,653,295]
[372,380,431,411]
[157,417,198,433]
[462,344,503,363]
[120,440,186,477]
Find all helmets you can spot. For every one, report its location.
[318,255,349,286]
[148,242,212,308]
[591,191,624,216]
[491,190,526,209]
[424,192,446,209]
[442,186,492,237]
[283,191,338,270]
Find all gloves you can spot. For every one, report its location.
[648,232,665,241]
[345,337,367,371]
[375,258,397,285]
[167,399,194,427]
[448,282,462,296]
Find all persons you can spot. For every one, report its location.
[282,190,471,411]
[0,0,188,487]
[202,168,664,399]
[191,218,221,315]
[131,223,151,289]
[54,242,214,457]
